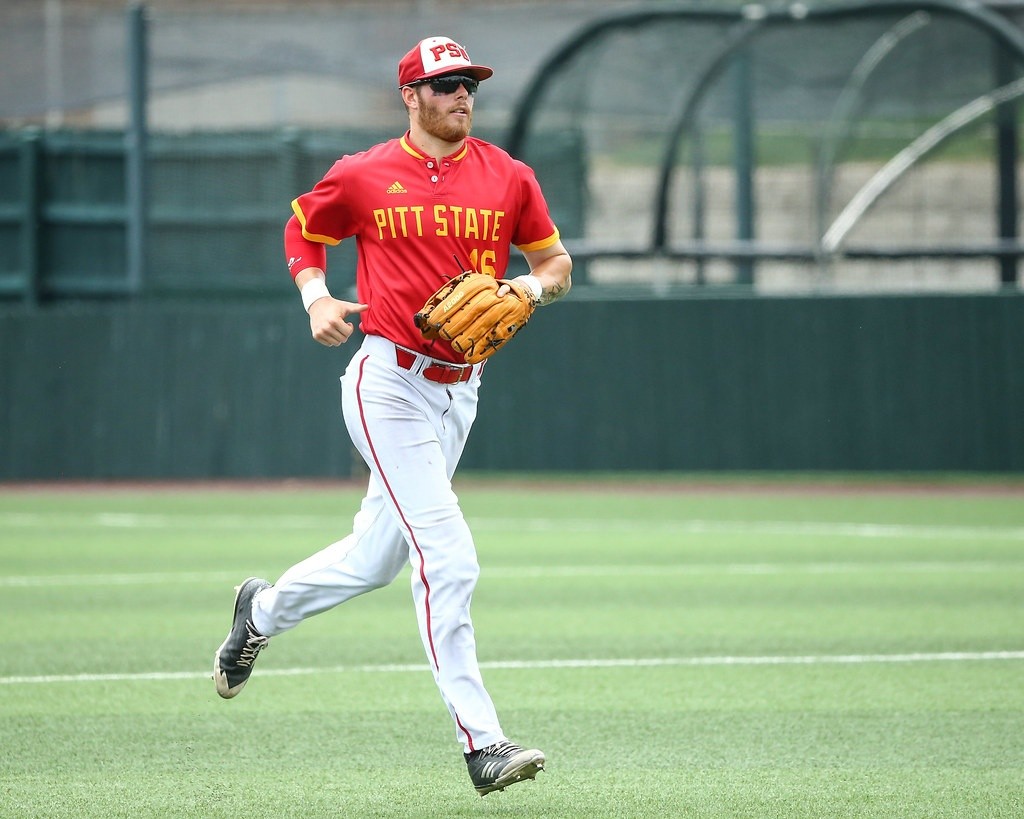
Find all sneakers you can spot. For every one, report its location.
[464,740,545,796]
[212,576,271,699]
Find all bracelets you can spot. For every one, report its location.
[300,279,332,313]
[515,274,542,301]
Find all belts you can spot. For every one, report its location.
[395,346,485,385]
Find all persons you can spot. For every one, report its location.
[211,36,571,797]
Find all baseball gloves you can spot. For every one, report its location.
[413,268,537,366]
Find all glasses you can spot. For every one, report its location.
[411,72,479,93]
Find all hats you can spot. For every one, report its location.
[398,36,493,85]
